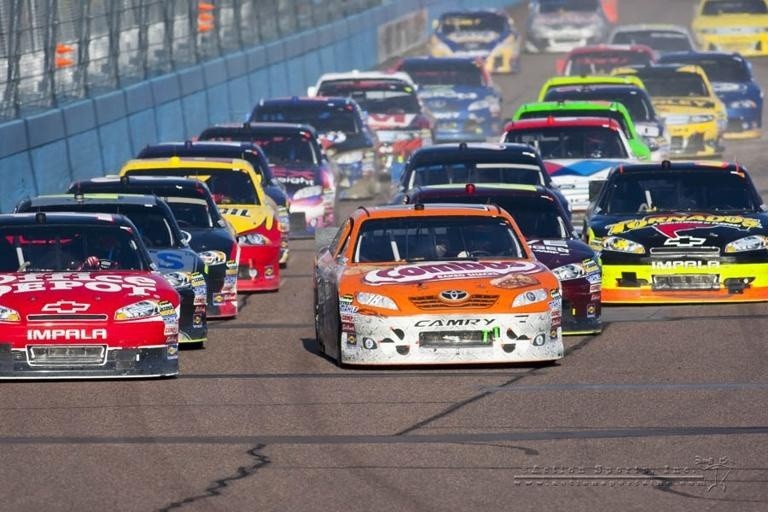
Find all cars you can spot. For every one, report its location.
[693,0,767,55]
[556,43,656,76]
[13,193,208,343]
[247,96,377,199]
[393,57,501,140]
[307,71,437,176]
[659,50,763,140]
[0,214,181,379]
[399,143,572,222]
[537,73,670,163]
[428,11,522,73]
[119,156,282,291]
[610,63,728,159]
[511,101,651,160]
[583,160,768,303]
[608,23,695,52]
[65,175,241,318]
[524,0,607,53]
[388,184,602,335]
[500,116,637,210]
[314,203,562,365]
[138,140,292,262]
[197,122,336,232]
